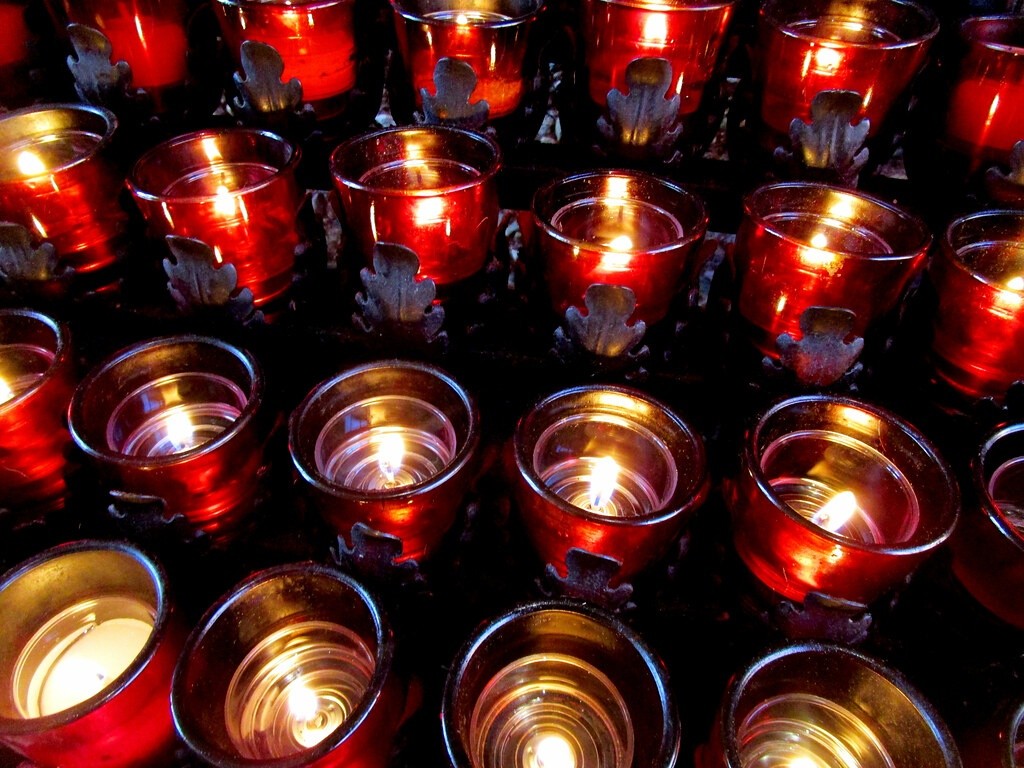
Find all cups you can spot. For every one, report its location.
[0,0,1024,768]
